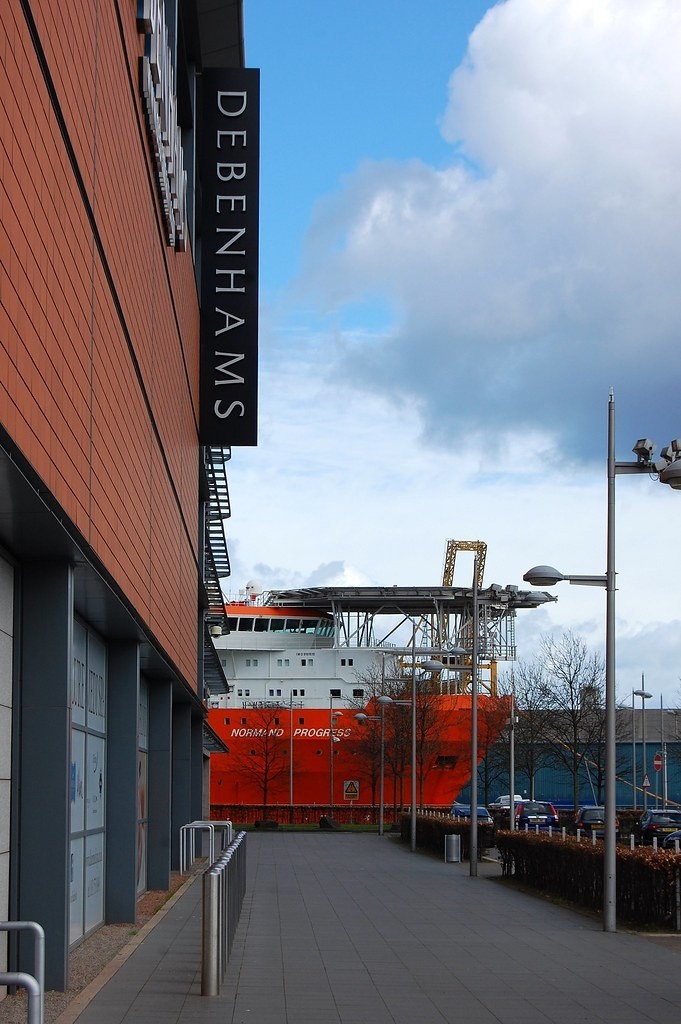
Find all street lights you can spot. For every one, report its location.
[522,565,619,933]
[280,645,480,874]
[613,689,677,814]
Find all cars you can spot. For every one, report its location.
[450,794,681,853]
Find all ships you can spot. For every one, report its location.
[206,577,560,832]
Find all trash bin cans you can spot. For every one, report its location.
[444,834,461,863]
